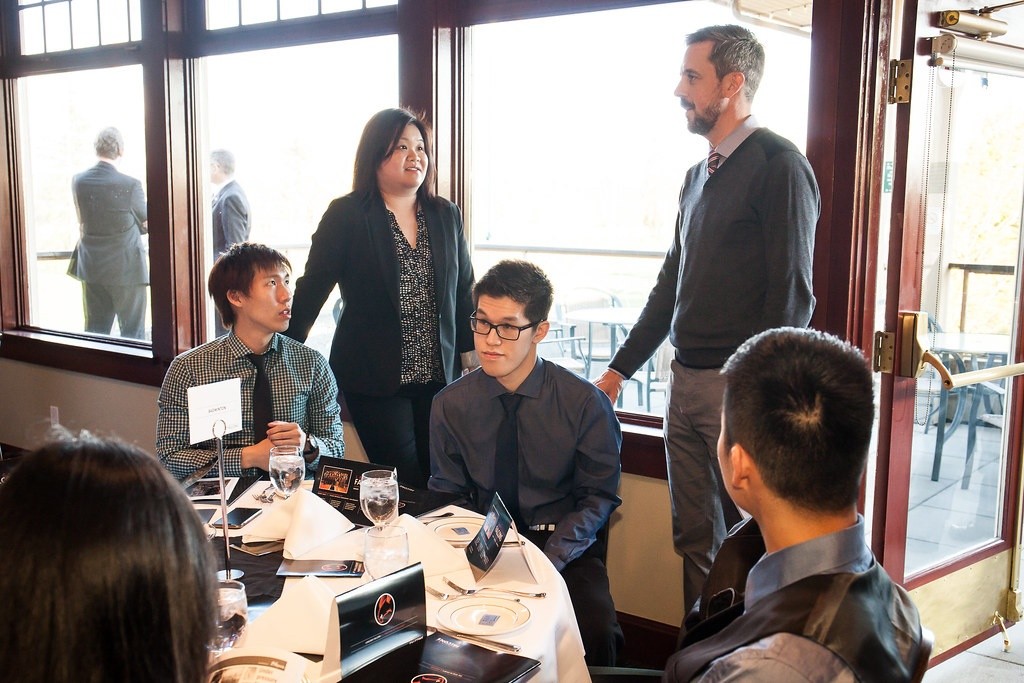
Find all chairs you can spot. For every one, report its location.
[535,328,675,414]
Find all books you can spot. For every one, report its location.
[212,506,263,530]
[229,541,284,556]
[194,509,217,522]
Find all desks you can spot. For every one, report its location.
[207,480,593,683]
[565,308,644,408]
[928,331,1009,489]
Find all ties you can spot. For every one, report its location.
[494,394,525,534]
[707,151,720,177]
[248,348,275,480]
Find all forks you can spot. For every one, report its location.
[251,483,276,504]
[425,576,547,602]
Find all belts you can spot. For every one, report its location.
[529,522,557,532]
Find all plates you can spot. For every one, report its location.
[438,597,531,637]
[425,515,487,542]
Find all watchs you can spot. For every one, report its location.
[303,433,318,455]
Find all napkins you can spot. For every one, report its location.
[386,513,468,578]
[281,484,355,558]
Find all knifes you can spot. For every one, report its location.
[427,627,522,653]
[453,541,526,548]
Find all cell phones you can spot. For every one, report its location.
[212,507,262,529]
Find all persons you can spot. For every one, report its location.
[278,104,480,489]
[157,242,346,480]
[663,327,922,683]
[0,419,222,683]
[428,258,628,683]
[591,24,821,652]
[211,148,252,339]
[66,128,148,340]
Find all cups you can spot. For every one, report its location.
[268,445,307,498]
[204,579,250,658]
[360,469,411,580]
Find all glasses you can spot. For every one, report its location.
[468,310,547,341]
